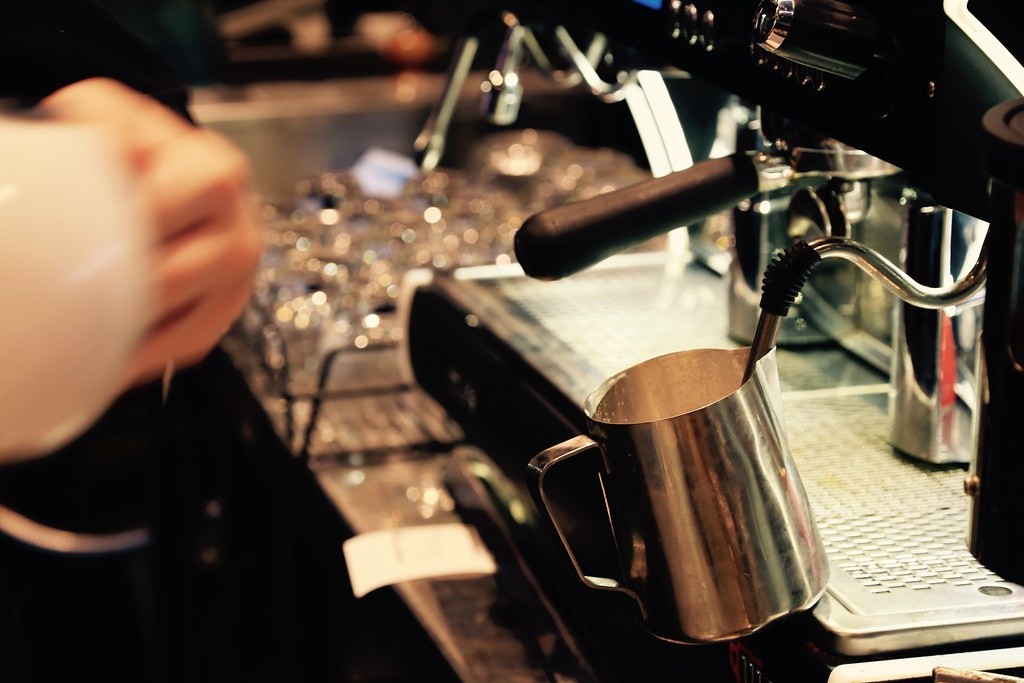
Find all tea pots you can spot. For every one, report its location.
[528,343,828,648]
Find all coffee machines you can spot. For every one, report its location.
[508,0,1024,594]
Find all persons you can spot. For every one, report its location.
[0,0,266,683]
